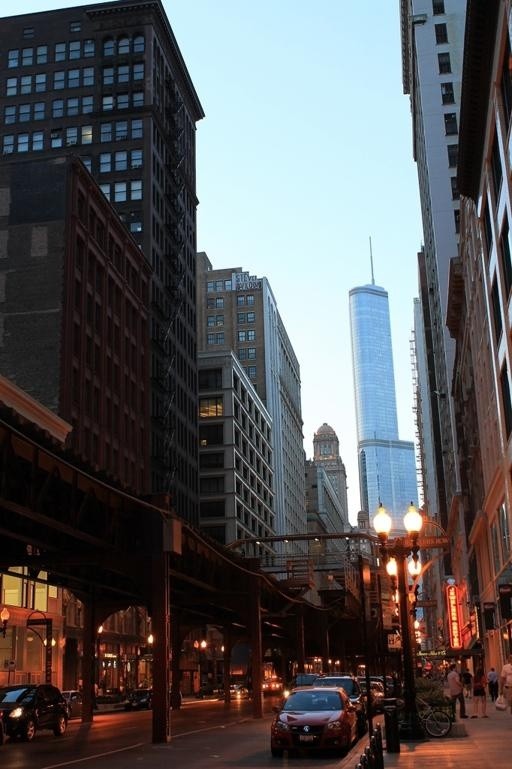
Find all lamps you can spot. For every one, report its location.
[1,606,10,639]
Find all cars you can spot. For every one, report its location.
[279,673,319,710]
[59,690,84,718]
[358,674,401,719]
[125,684,183,711]
[228,682,253,699]
[262,676,284,698]
[270,686,358,758]
[311,671,367,738]
[1,683,70,741]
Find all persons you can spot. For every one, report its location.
[443,655,512,719]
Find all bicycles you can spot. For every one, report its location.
[397,692,452,739]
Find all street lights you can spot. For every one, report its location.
[372,500,423,740]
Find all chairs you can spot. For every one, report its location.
[296,694,340,706]
